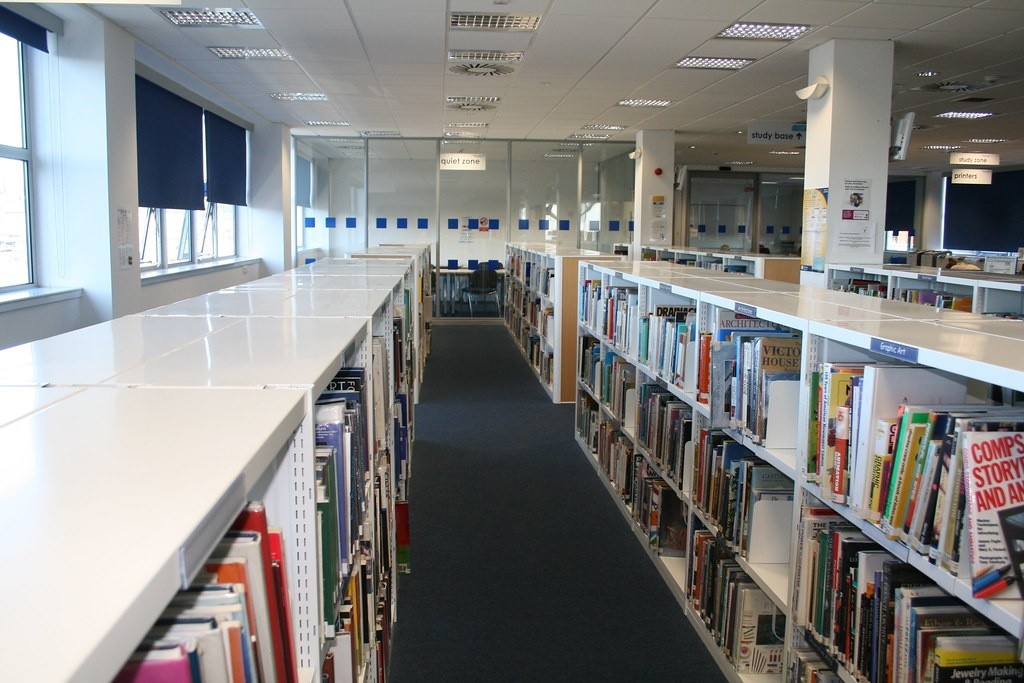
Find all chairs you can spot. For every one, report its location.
[461,267,501,318]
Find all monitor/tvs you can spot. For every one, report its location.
[889,112,915,160]
[675,165,687,191]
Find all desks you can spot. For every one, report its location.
[431,268,504,317]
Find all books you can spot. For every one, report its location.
[806,336,1024,630]
[692,421,795,565]
[795,500,1024,683]
[786,642,846,683]
[562,263,696,558]
[683,517,787,675]
[695,306,804,450]
[614,237,1024,321]
[112,254,435,682]
[495,244,560,391]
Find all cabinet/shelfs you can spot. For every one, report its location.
[907,248,1024,276]
[1,255,416,683]
[613,241,633,263]
[504,241,628,404]
[343,241,431,383]
[575,259,1024,682]
[640,245,800,282]
[824,263,1024,322]
